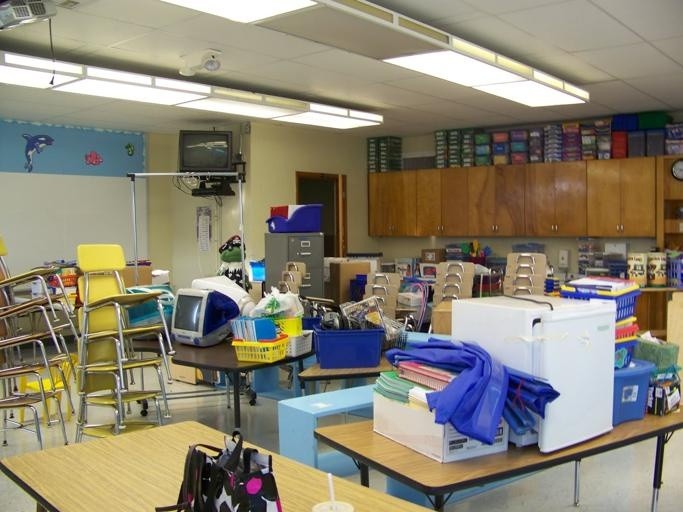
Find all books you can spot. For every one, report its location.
[565,275,629,291]
[231,317,278,341]
[373,360,462,413]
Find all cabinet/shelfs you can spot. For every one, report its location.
[417,165,467,240]
[467,161,525,241]
[655,155,683,253]
[633,287,678,334]
[274,383,377,478]
[263,228,327,300]
[382,470,543,509]
[586,154,656,240]
[367,168,417,240]
[525,159,586,241]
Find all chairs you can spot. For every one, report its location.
[360,251,550,335]
[0,228,178,452]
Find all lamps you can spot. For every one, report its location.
[0,44,387,134]
[152,0,593,112]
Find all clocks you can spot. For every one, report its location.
[669,156,682,187]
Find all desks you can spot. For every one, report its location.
[0,416,437,510]
[312,410,683,510]
[296,355,396,401]
[122,332,322,429]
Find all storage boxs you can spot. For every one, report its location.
[314,328,387,371]
[326,261,371,308]
[612,357,660,428]
[402,151,436,171]
[263,200,325,232]
[370,388,511,468]
[420,249,446,262]
[248,261,265,282]
[365,135,401,173]
[436,110,683,168]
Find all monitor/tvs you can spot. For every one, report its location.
[171,288,241,349]
[177,130,233,172]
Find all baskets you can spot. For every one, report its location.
[665,258,683,290]
[273,317,303,337]
[544,274,640,372]
[288,328,314,359]
[46,267,79,288]
[228,332,289,365]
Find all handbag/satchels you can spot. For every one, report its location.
[154,430,285,512]
